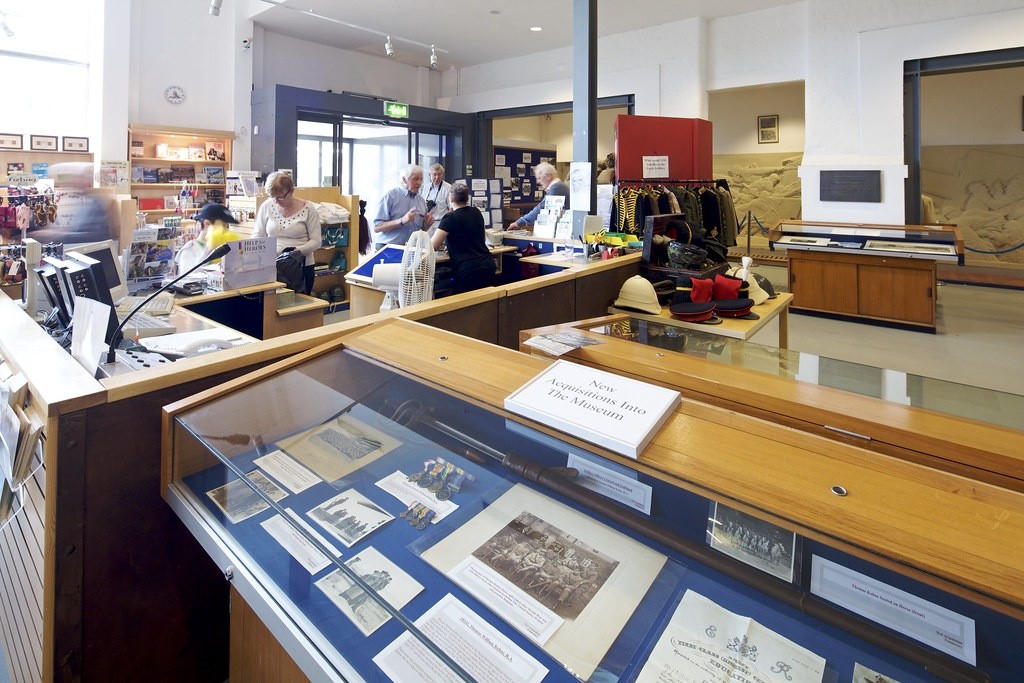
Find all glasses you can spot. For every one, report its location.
[269,189,291,200]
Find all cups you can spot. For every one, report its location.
[598,245,607,254]
[582,243,590,257]
[564,235,574,256]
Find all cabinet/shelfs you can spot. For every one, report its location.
[228,186,359,315]
[128,123,239,225]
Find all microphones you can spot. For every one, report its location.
[106,244,233,364]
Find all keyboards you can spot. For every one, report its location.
[116,312,176,340]
[116,295,177,315]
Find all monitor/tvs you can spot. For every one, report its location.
[344,244,430,286]
[33,239,129,348]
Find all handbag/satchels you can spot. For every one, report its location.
[322,222,348,246]
[329,284,345,301]
[276,247,306,293]
[330,250,347,273]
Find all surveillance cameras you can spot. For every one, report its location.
[243,37,252,45]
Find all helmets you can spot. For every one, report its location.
[752,272,777,299]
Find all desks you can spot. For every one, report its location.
[519,252,605,268]
[606,291,794,378]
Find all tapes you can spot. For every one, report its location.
[607,247,614,255]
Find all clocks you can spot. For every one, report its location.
[164,86,185,104]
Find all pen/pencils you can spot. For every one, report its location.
[135,277,164,282]
[586,241,587,244]
[603,240,609,246]
[227,337,242,341]
[579,235,583,243]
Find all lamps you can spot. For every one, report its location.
[384,36,394,56]
[209,0,222,16]
[429,44,438,68]
[758,115,779,144]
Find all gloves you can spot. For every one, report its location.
[290,249,303,264]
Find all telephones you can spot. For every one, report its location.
[146,337,232,362]
[152,282,162,288]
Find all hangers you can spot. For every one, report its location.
[622,179,720,193]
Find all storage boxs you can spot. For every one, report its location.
[641,213,729,284]
[207,236,278,292]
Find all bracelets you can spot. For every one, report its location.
[399,218,404,227]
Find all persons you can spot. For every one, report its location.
[431,183,499,295]
[249,171,322,296]
[374,164,436,254]
[597,153,615,183]
[173,203,241,277]
[508,162,570,230]
[422,163,454,238]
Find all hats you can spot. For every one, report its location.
[716,309,760,320]
[659,241,708,270]
[702,239,732,268]
[725,267,769,305]
[677,313,723,324]
[669,275,716,314]
[201,204,239,223]
[712,273,755,311]
[614,274,661,314]
